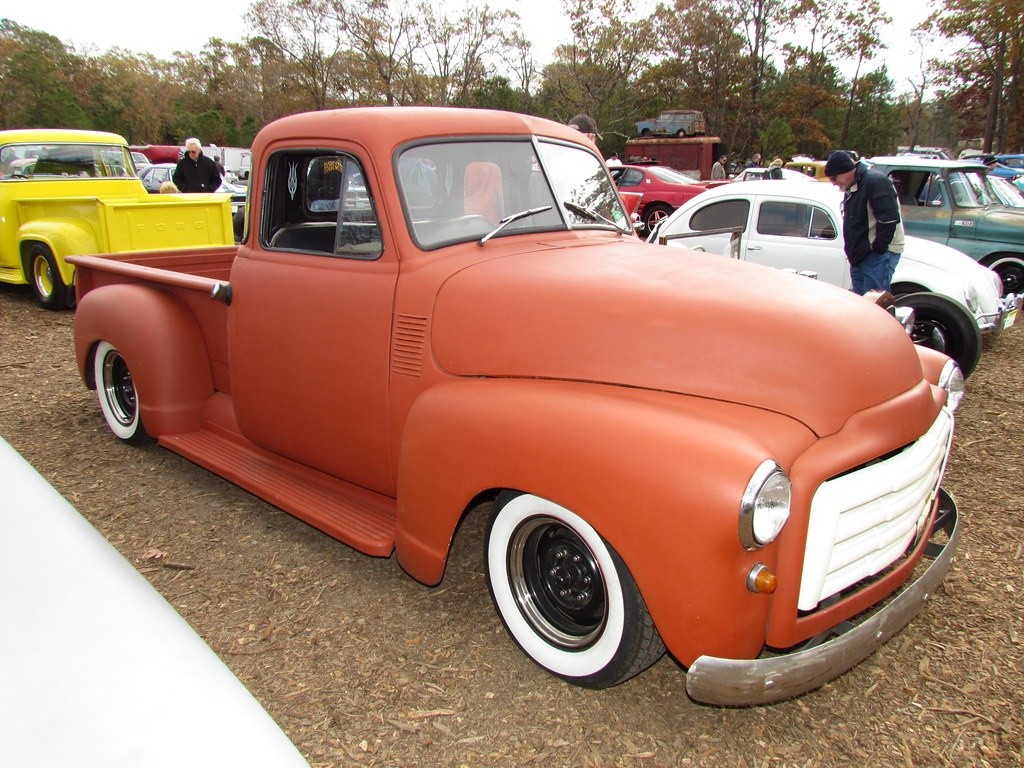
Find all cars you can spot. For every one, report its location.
[644,179,1020,339]
[101,151,250,216]
[735,146,1024,299]
[632,112,706,136]
[608,161,732,238]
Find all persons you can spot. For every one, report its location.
[763,159,784,180]
[710,154,728,179]
[172,137,222,193]
[824,149,905,296]
[565,115,603,145]
[744,154,761,168]
[982,155,999,168]
[307,156,348,212]
[159,181,178,193]
[212,156,225,177]
[605,151,636,182]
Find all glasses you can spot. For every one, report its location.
[186,150,198,154]
[829,175,837,184]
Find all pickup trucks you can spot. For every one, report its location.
[63,106,964,710]
[0,130,235,311]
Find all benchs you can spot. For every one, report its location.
[901,196,918,205]
[269,219,453,255]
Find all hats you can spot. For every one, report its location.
[568,114,603,140]
[983,156,998,165]
[825,152,855,177]
